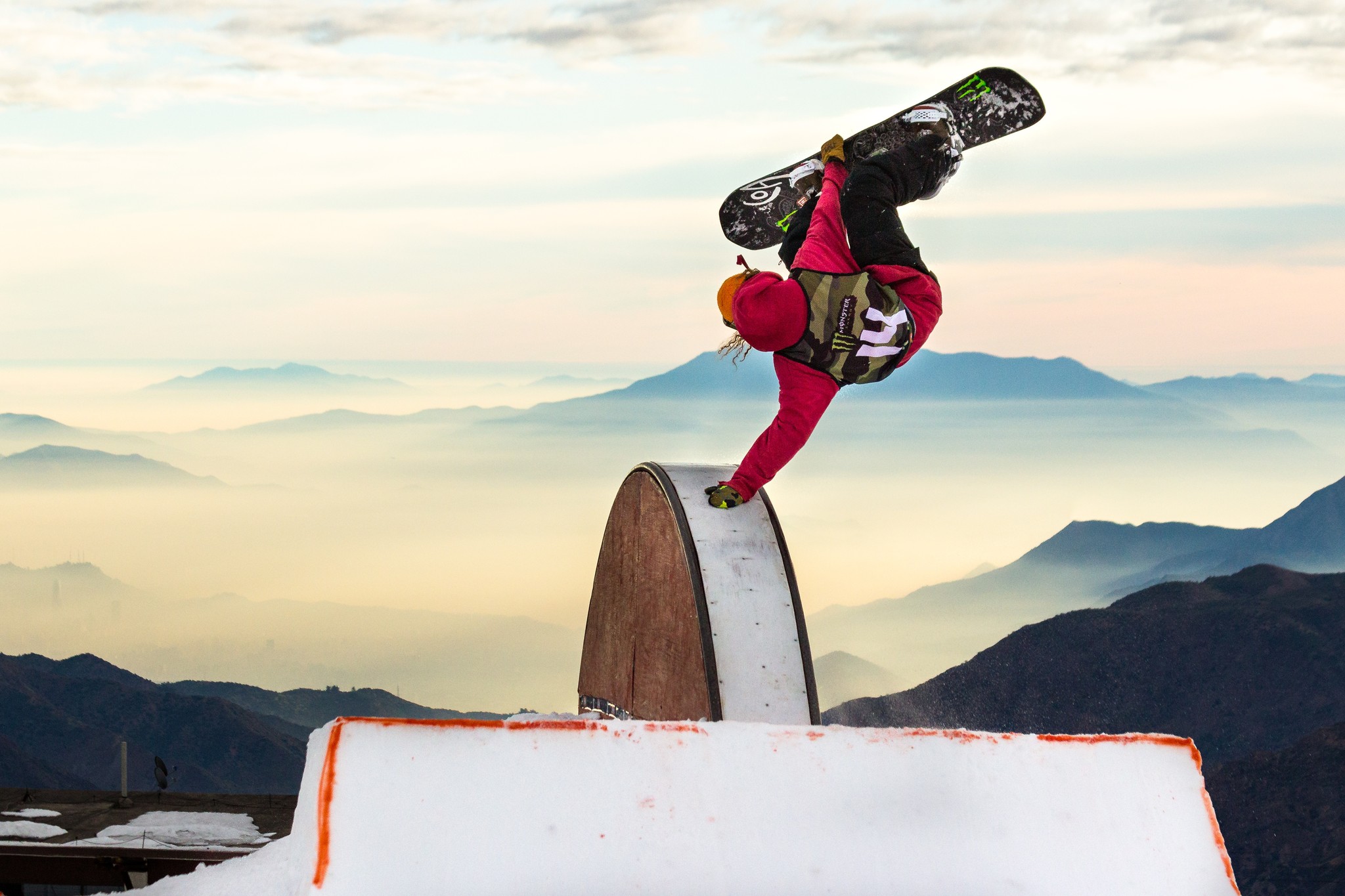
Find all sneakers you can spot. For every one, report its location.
[909,104,960,200]
[793,163,823,198]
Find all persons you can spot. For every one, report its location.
[703,103,967,508]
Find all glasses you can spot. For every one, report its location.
[722,316,738,331]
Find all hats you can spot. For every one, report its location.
[717,274,747,326]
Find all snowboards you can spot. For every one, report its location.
[719,67,1046,253]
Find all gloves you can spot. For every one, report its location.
[821,134,845,166]
[704,485,743,509]
